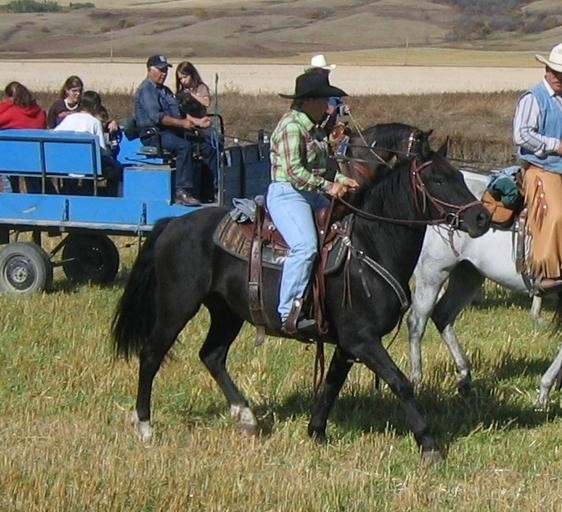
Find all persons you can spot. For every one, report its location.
[303,54,352,135]
[135,55,219,206]
[0,76,123,196]
[513,44,562,289]
[266,74,360,331]
[175,61,210,118]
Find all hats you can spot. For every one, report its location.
[145,54,173,71]
[302,54,337,72]
[276,72,349,100]
[533,42,562,74]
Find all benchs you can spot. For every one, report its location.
[117,113,223,167]
[0,129,106,196]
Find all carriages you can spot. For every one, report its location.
[1,110,562,318]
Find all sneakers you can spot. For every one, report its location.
[278,316,318,337]
[533,276,562,298]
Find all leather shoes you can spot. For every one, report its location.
[173,188,201,208]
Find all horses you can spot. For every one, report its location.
[407,168,562,414]
[335,122,435,186]
[110,135,492,464]
